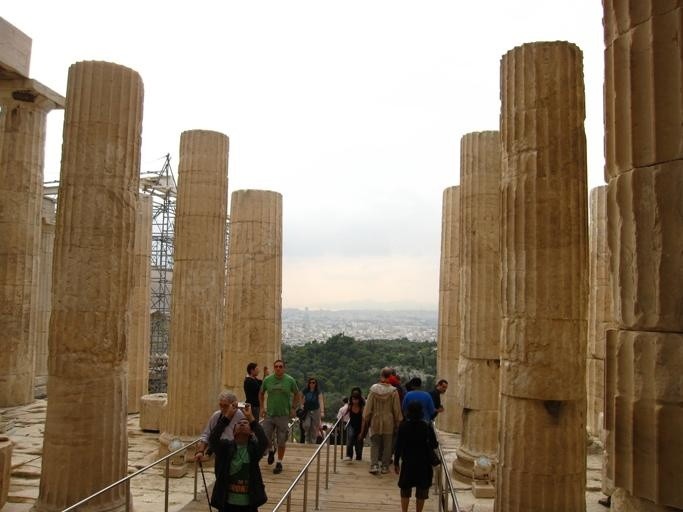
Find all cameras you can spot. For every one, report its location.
[237,401,246,408]
[288,417,301,429]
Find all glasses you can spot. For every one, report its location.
[274,365,282,368]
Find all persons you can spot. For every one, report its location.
[195,360,448,512]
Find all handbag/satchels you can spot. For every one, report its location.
[296,408,305,420]
[425,422,440,466]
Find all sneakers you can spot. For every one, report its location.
[272,461,282,474]
[267,446,275,465]
[343,457,351,461]
[368,463,378,472]
[381,464,388,473]
